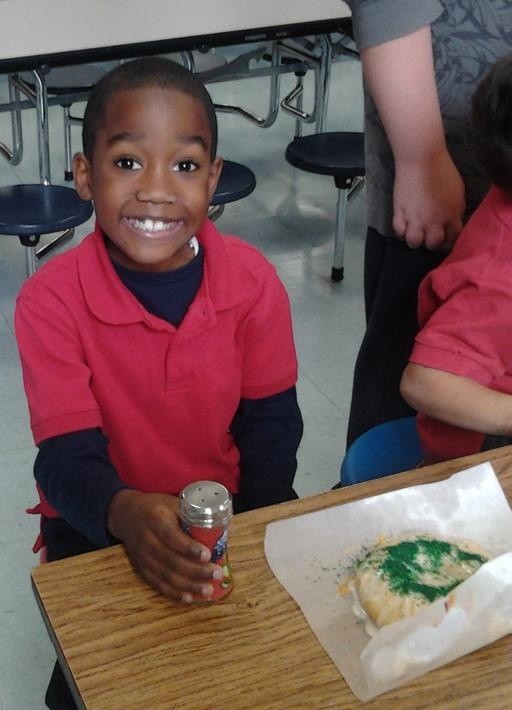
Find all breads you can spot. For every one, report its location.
[352,529,494,636]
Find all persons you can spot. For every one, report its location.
[399,50,512,464]
[330,0,512,495]
[13,55,306,709]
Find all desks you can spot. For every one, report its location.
[0,0,355,183]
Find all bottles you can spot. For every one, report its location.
[173,477,238,606]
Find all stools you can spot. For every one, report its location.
[0,50,367,284]
[339,418,431,488]
